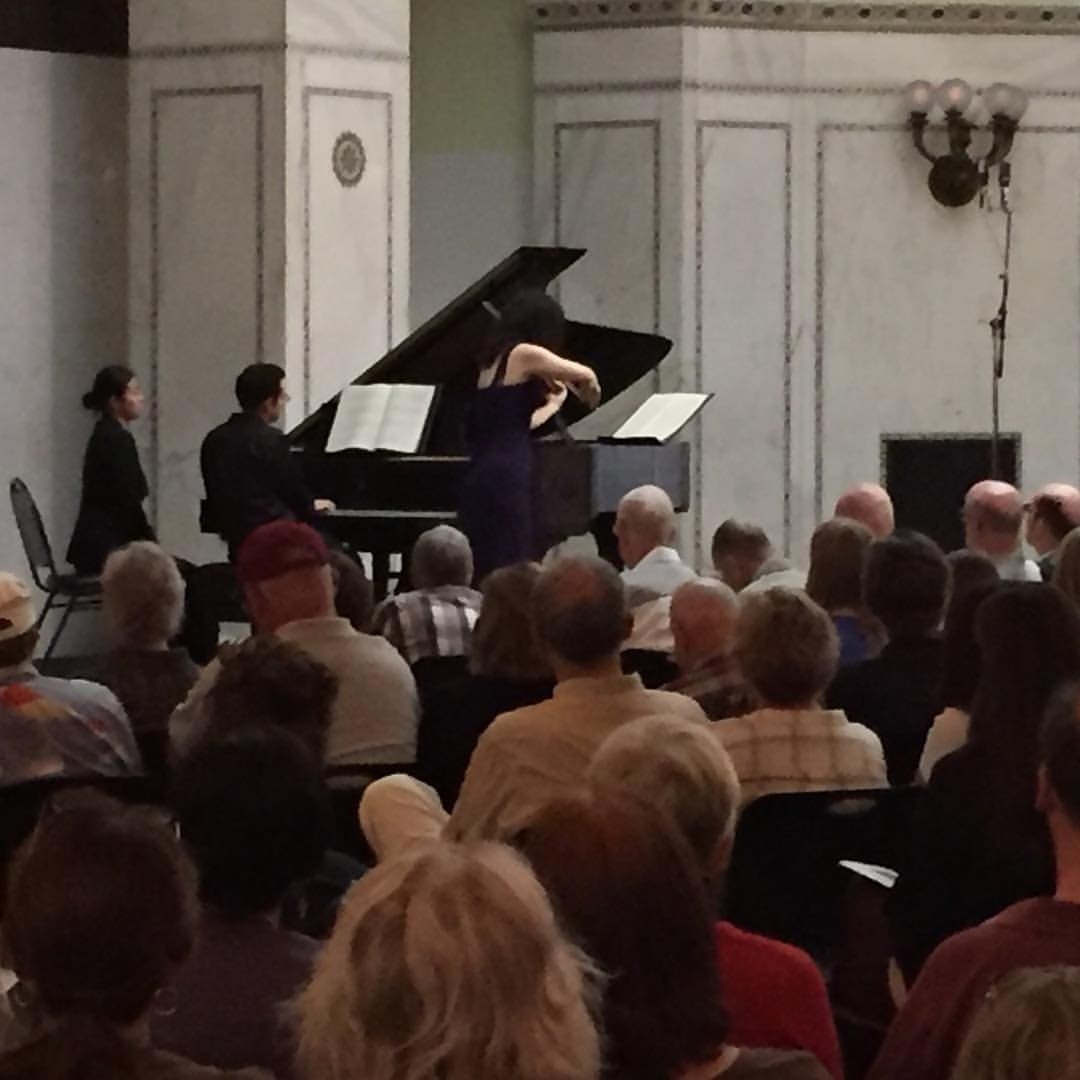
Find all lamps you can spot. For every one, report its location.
[905,79,1027,208]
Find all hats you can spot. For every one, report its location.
[0,571,37,640]
[238,519,330,584]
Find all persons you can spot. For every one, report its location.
[455,287,601,592]
[65,366,218,666]
[200,362,373,630]
[1,480,1080,1080]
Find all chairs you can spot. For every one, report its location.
[726,788,924,925]
[10,477,103,674]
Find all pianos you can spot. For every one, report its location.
[196,245,694,600]
